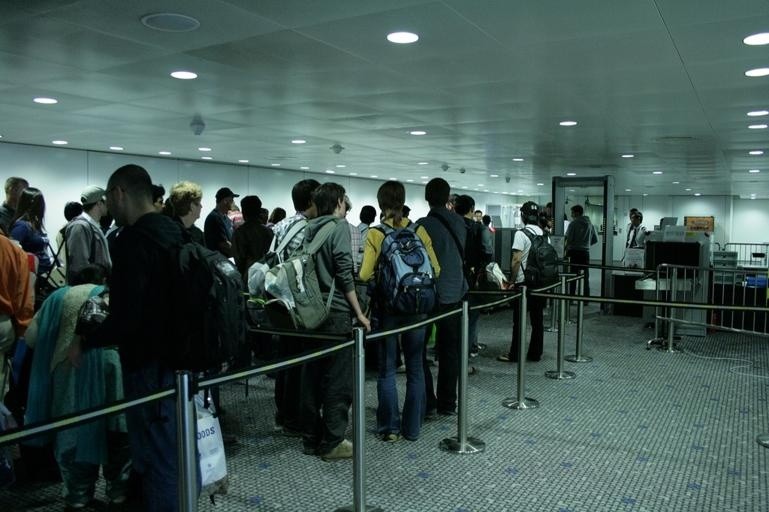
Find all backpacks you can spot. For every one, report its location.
[264,217,347,332]
[131,215,244,374]
[518,227,559,293]
[370,216,436,319]
[244,215,309,329]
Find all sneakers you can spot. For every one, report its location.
[380,341,491,375]
[376,405,458,443]
[63,486,132,510]
[496,354,518,362]
[302,438,353,462]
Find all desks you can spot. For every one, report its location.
[611,271,647,318]
[708,281,768,334]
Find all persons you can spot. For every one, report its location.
[620,212,651,261]
[625,208,639,234]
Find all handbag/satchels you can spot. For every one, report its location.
[464,261,509,313]
[193,372,229,496]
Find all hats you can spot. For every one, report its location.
[80,185,107,206]
[216,187,240,203]
[519,200,540,216]
